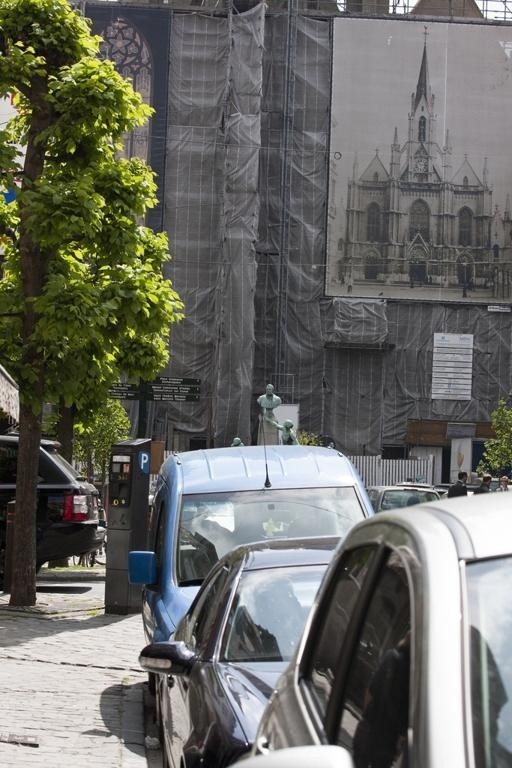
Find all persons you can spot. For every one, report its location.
[495,476,508,492]
[231,437,244,447]
[263,415,300,445]
[255,383,280,409]
[446,470,467,499]
[471,473,492,495]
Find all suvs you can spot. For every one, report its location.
[1,433,107,587]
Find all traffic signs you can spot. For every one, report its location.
[152,385,200,394]
[106,382,138,391]
[107,392,140,400]
[152,395,200,403]
[152,375,200,385]
